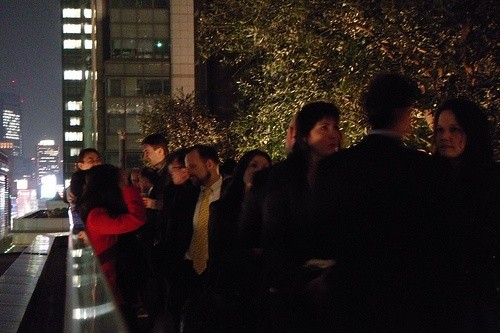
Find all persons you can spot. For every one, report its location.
[67,72,500,333]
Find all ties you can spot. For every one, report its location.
[193,188,212,276]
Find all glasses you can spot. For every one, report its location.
[169,166,186,171]
